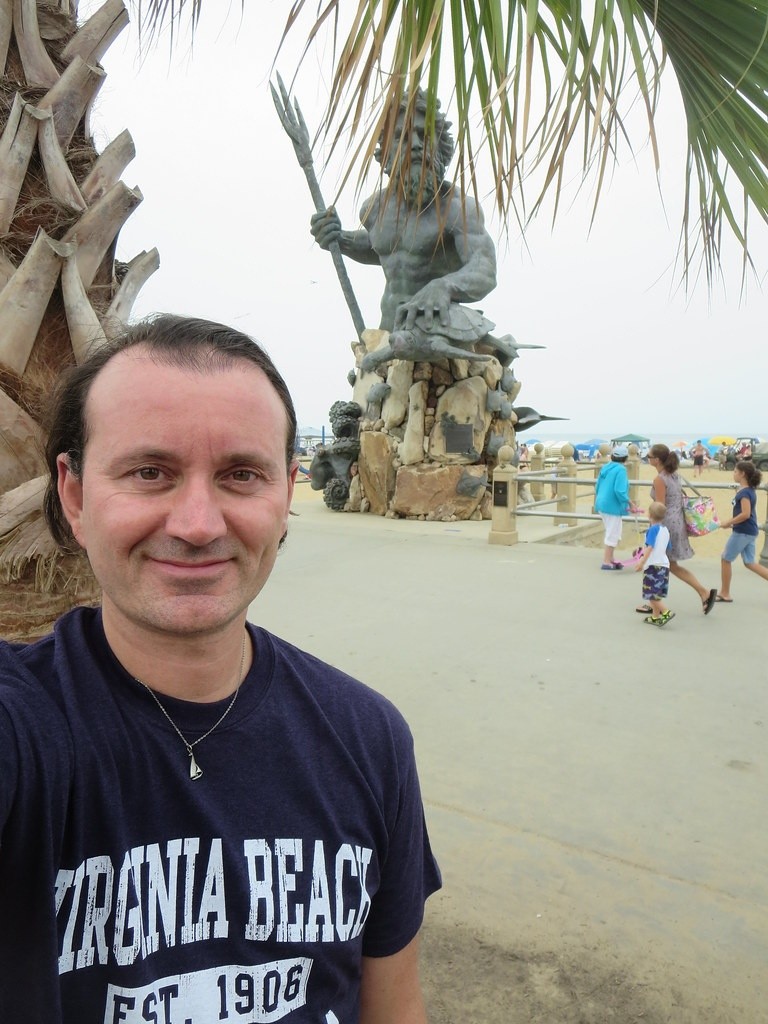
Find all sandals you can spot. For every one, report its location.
[643,616,661,626]
[659,610,675,627]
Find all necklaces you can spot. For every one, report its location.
[132,628,245,783]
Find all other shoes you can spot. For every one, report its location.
[601,561,623,570]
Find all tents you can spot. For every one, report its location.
[520,439,610,459]
[611,434,651,455]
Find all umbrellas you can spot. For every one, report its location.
[709,436,736,447]
[671,441,688,460]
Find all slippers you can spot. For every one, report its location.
[715,596,732,602]
[702,589,717,615]
[636,605,653,613]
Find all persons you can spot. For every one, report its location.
[636,444,717,615]
[635,502,675,626]
[0,312,444,1022]
[309,86,495,330]
[520,444,528,469]
[681,439,752,477]
[594,446,638,571]
[682,444,710,464]
[714,461,768,602]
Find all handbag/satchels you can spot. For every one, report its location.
[681,476,721,537]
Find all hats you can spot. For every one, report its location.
[612,446,629,458]
